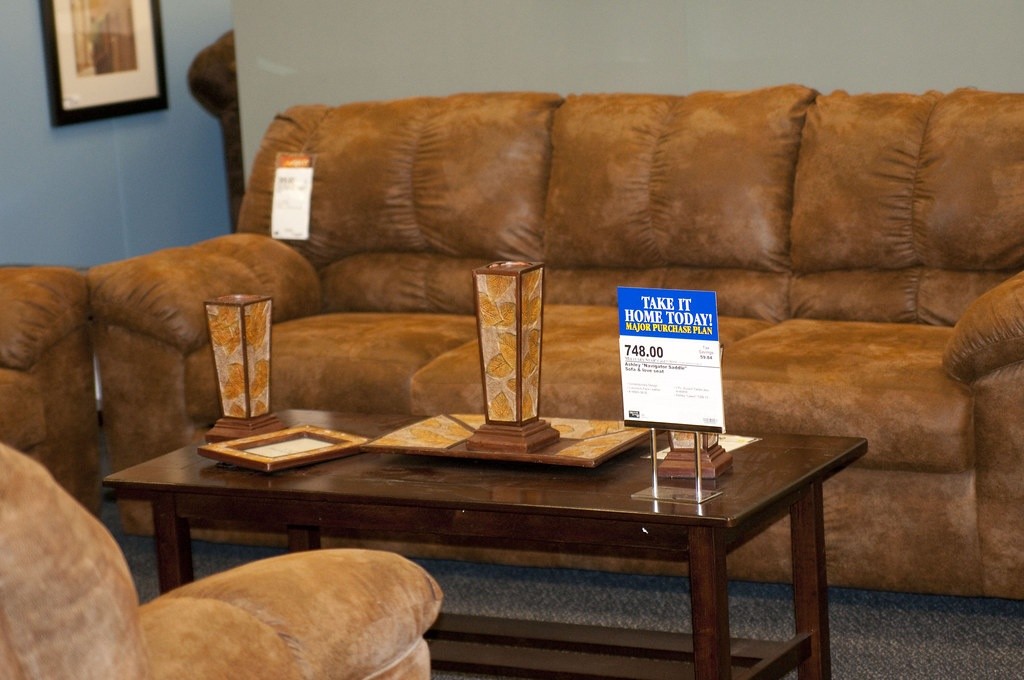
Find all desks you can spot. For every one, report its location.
[101,408,870,680]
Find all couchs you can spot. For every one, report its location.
[83,86,1024,600]
[0,266,441,680]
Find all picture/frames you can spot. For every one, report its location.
[40,0,169,128]
[194,423,372,473]
